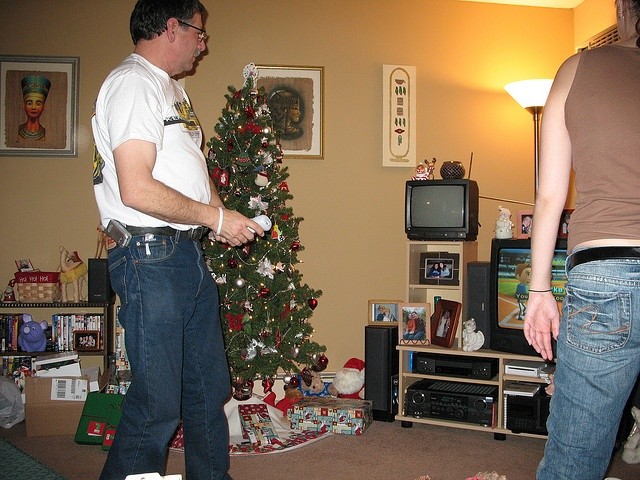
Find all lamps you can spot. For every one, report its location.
[504,79,554,204]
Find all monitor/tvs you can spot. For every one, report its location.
[491,238,567,358]
[405,179,481,240]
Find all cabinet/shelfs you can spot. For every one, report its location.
[396,241,619,452]
[0,301,115,370]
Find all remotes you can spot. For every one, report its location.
[245,214,272,233]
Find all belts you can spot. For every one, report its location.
[125,225,211,240]
[566,246,640,271]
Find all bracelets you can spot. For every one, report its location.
[216,207,224,234]
[529,289,552,292]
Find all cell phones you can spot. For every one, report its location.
[97,219,133,248]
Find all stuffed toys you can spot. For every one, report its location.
[331,357,365,399]
[274,384,304,415]
[56,245,86,301]
[291,368,338,398]
[1,279,13,303]
[462,318,484,352]
[18,312,48,351]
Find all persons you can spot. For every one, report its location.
[404,311,425,340]
[525,0,640,480]
[76,335,95,348]
[515,262,531,320]
[522,216,532,233]
[435,311,450,338]
[15,74,52,141]
[427,262,450,278]
[267,86,306,139]
[91,1,264,476]
[377,305,396,322]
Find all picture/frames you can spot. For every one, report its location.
[398,303,431,347]
[431,299,461,348]
[0,55,81,157]
[73,330,100,352]
[368,299,403,327]
[253,65,324,160]
[517,210,534,240]
[558,209,577,238]
[420,252,459,286]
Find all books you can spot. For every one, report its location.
[0,312,24,354]
[51,313,104,350]
[1,354,32,393]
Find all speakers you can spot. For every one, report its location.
[88,258,117,304]
[364,324,398,422]
[466,260,490,350]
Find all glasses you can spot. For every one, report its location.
[178,20,209,42]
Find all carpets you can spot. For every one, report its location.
[1,438,70,480]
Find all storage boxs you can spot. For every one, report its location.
[25,369,110,437]
